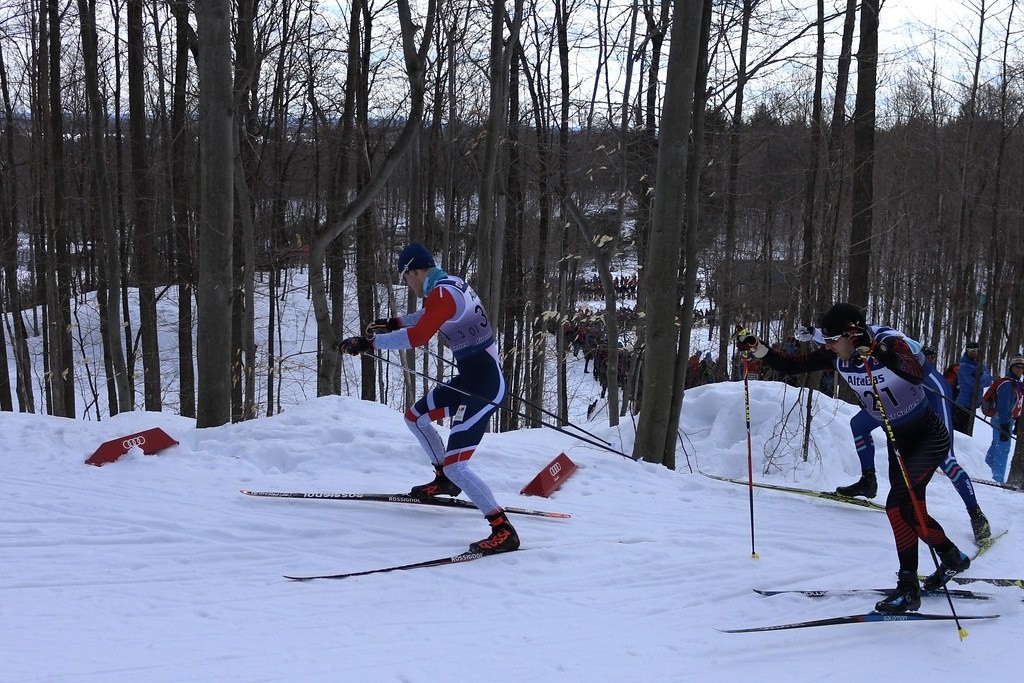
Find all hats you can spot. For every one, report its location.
[966,342,980,353]
[819,303,869,336]
[398,242,436,273]
[1007,353,1024,371]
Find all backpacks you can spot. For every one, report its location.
[943,362,960,401]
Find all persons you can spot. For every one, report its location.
[685,350,731,389]
[694,308,720,328]
[736,305,787,324]
[618,343,629,391]
[794,325,991,546]
[576,275,605,301]
[951,342,995,437]
[985,353,1024,482]
[731,304,970,613]
[613,273,637,300]
[732,336,834,397]
[547,307,608,399]
[339,242,520,554]
[922,345,938,367]
[695,277,702,293]
[616,304,637,330]
[942,363,960,401]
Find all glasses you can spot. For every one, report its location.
[967,348,980,352]
[1013,365,1024,370]
[399,258,414,285]
[822,331,849,344]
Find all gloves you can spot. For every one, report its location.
[844,326,877,355]
[732,330,771,359]
[339,334,375,356]
[366,317,400,334]
[1000,422,1011,442]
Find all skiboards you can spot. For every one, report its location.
[936,470,1022,492]
[240,489,572,580]
[699,468,1009,561]
[895,570,1024,590]
[710,587,1024,633]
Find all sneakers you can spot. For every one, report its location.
[836,468,878,499]
[968,505,991,545]
[411,462,462,497]
[469,509,520,555]
[925,542,970,591]
[874,571,921,614]
